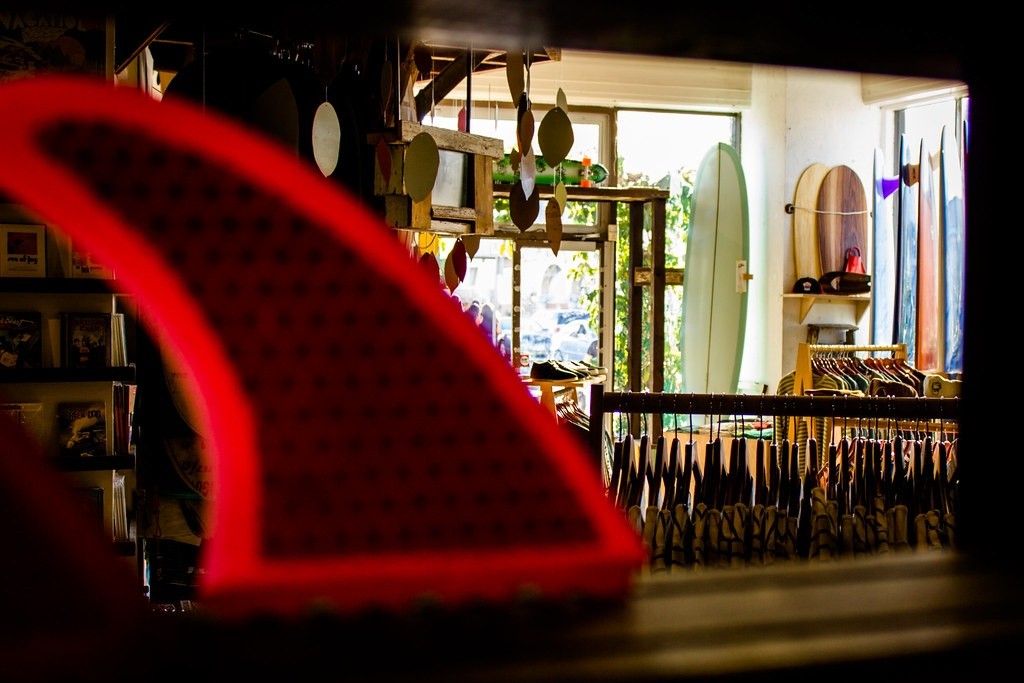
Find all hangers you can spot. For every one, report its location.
[793,343,916,378]
[555,399,583,425]
[608,387,959,549]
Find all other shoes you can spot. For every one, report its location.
[529,355,609,383]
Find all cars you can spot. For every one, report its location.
[498,309,598,362]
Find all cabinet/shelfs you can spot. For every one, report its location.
[1,275,138,555]
